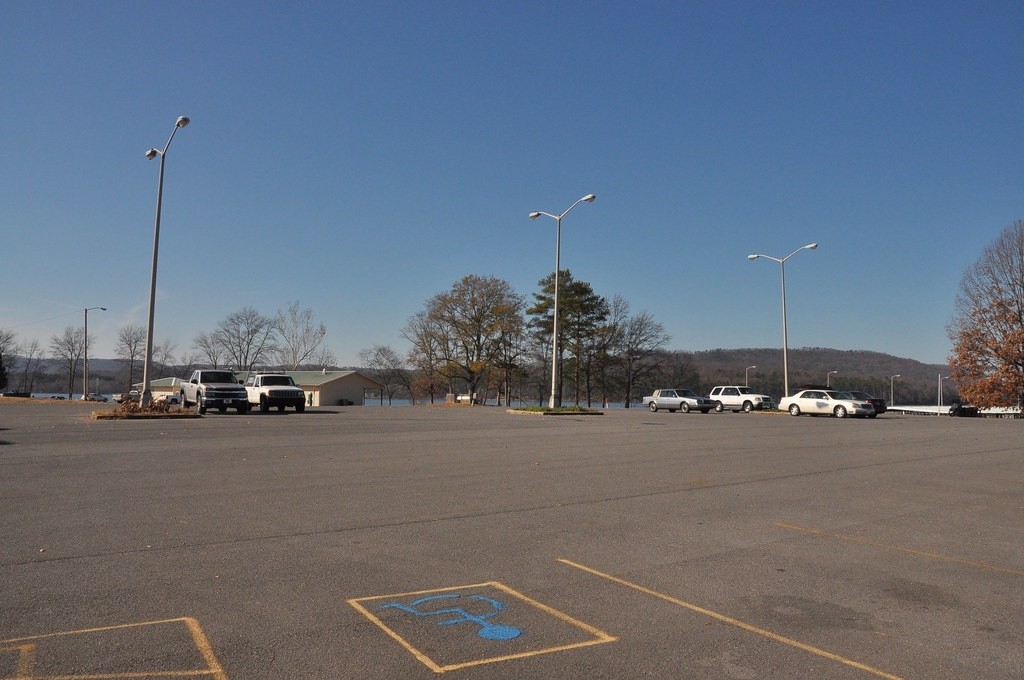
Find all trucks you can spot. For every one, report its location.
[112,390,141,403]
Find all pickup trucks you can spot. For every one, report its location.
[242,373,306,414]
[180,369,249,415]
[157,390,181,404]
[1,390,31,397]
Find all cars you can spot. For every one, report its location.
[850,390,887,418]
[777,390,876,418]
[641,388,719,413]
[81,393,108,402]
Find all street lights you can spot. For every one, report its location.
[529,193,596,412]
[826,371,838,388]
[941,376,949,408]
[83,307,107,402]
[891,374,901,408]
[746,366,756,387]
[746,242,819,397]
[137,114,190,412]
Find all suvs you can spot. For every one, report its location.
[708,385,771,413]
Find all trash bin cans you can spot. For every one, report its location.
[340,399,347,405]
